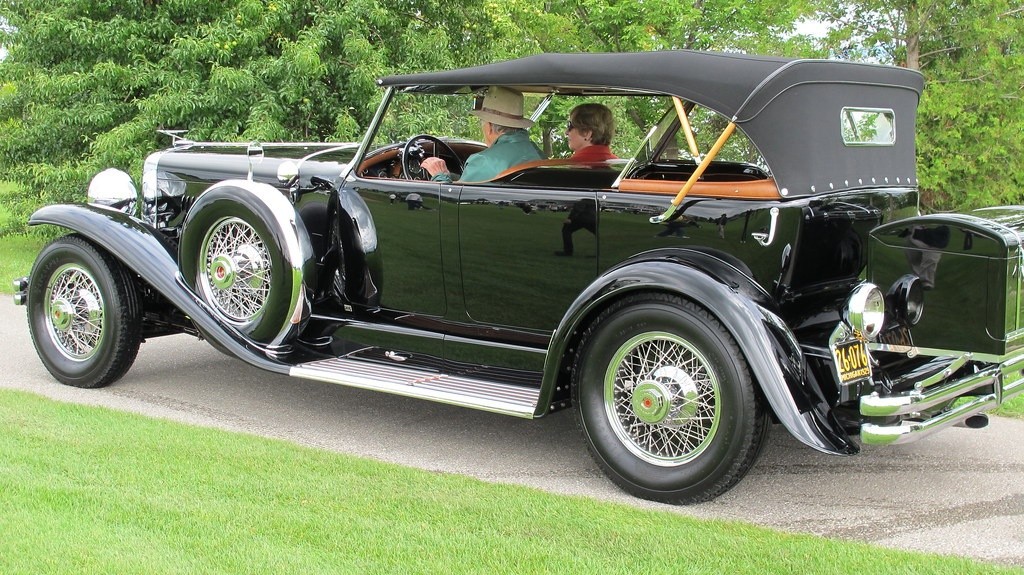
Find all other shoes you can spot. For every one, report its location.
[555,249,573,257]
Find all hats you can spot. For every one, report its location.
[470,86,533,130]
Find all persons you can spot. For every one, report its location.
[653,222,685,239]
[564,102,618,158]
[496,200,538,217]
[388,192,396,206]
[896,221,952,290]
[553,198,599,256]
[418,85,548,182]
[715,213,727,240]
[405,192,425,211]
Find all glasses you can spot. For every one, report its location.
[568,120,574,131]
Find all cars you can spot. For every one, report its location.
[23,49,1024,507]
